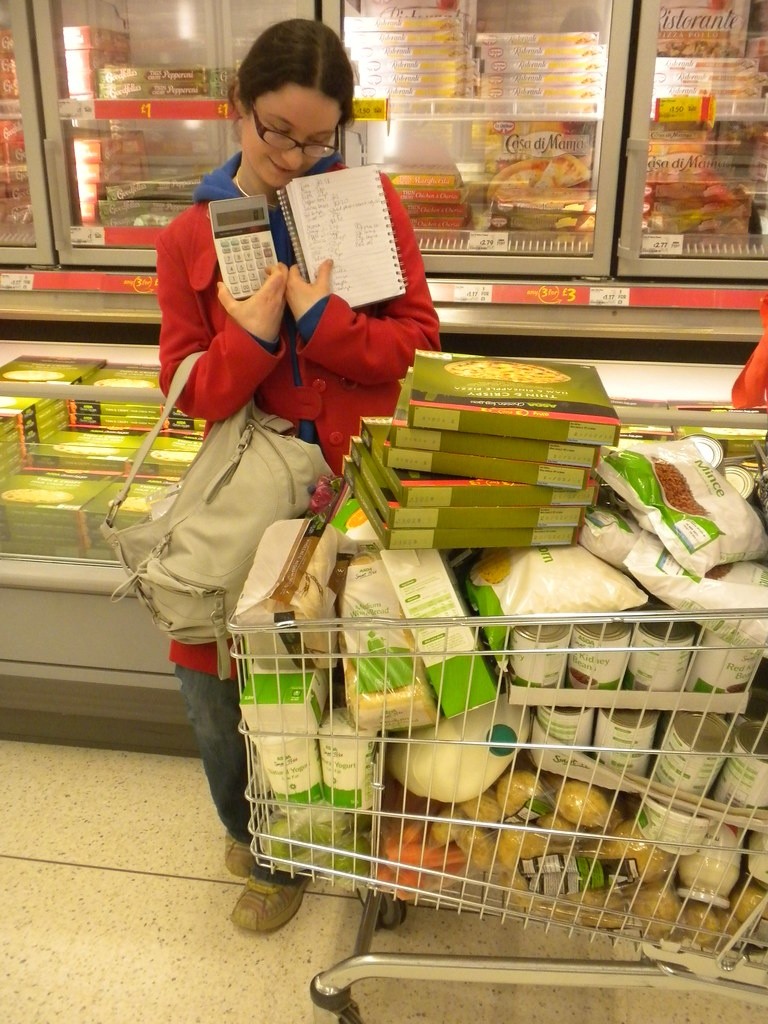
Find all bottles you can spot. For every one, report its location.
[387,693,532,803]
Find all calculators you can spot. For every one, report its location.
[208,193,279,299]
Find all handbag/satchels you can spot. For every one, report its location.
[100,350,338,644]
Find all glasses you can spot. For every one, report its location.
[250,99,340,157]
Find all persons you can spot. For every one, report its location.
[156,18,442,931]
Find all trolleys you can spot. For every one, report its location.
[228,606,768,1021]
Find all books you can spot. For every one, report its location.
[274,163,409,306]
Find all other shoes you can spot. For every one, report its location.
[225,830,319,931]
